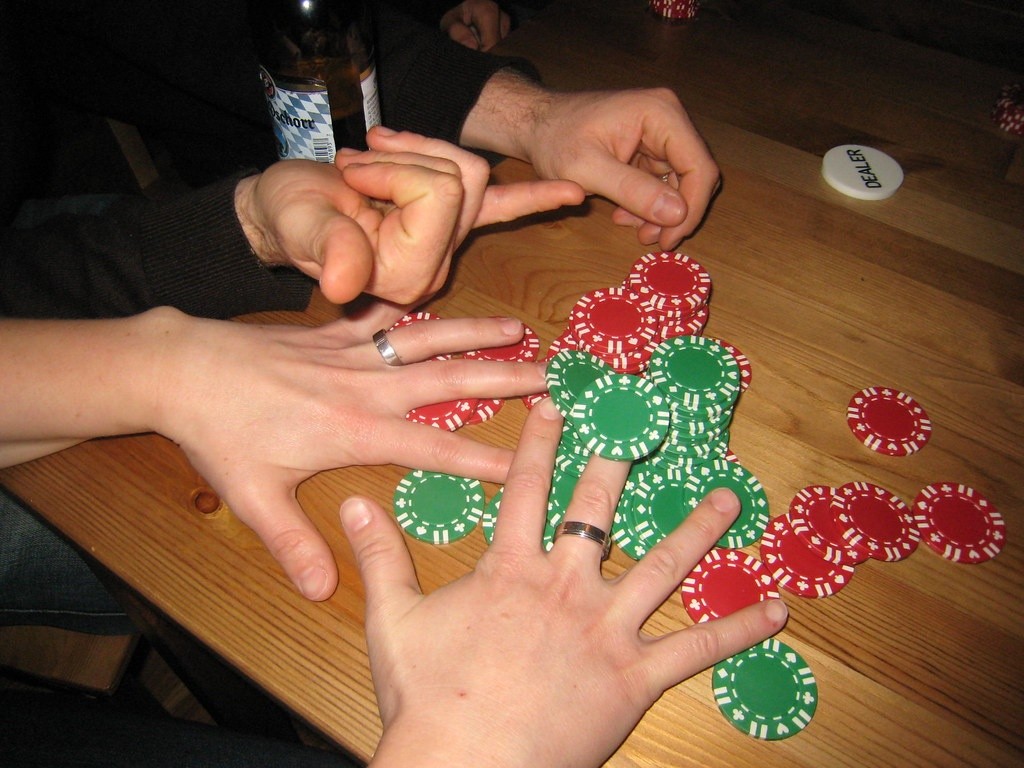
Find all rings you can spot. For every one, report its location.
[552,521,610,562]
[373,329,402,366]
[662,175,668,182]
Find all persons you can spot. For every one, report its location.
[392,0,511,53]
[1,0,721,317]
[1,296,788,768]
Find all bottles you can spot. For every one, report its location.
[250,0,382,165]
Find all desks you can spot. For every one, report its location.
[0,0,1024,768]
